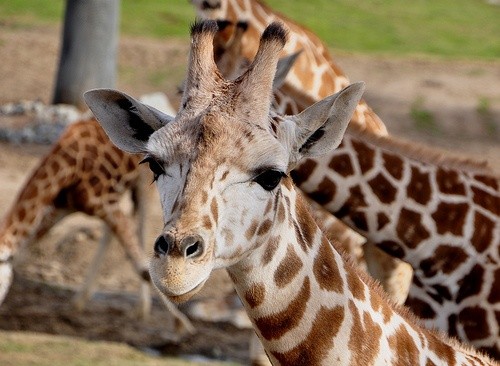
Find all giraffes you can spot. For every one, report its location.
[0,0,499,366]
